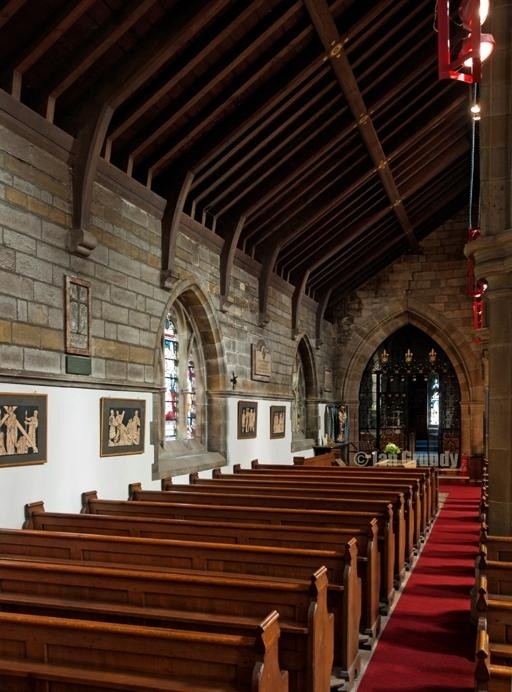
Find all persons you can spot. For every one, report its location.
[337,405,346,442]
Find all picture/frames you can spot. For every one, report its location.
[99,396,147,458]
[269,405,286,440]
[1,392,49,468]
[236,400,258,440]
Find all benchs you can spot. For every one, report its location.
[471,522,511,689]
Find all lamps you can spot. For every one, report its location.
[452,0,495,69]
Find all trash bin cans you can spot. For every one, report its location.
[469,455,485,481]
